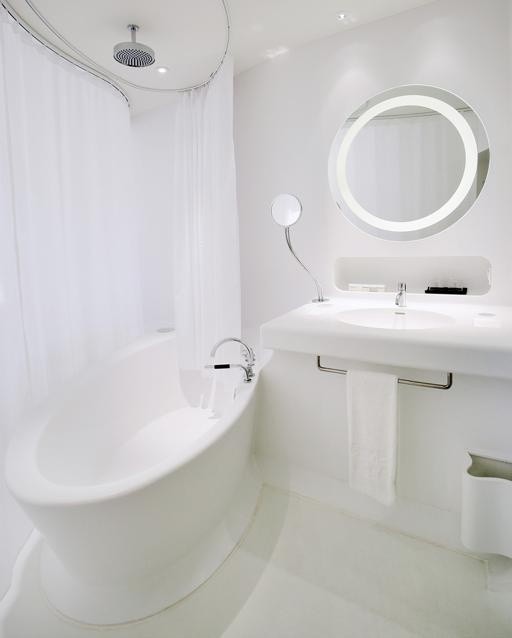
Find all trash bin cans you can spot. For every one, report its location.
[461,450,512,559]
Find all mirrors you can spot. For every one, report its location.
[269,192,328,304]
[326,82,491,244]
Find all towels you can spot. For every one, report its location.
[344,368,398,508]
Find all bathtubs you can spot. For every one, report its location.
[1,325,276,625]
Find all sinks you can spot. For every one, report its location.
[261,291,511,378]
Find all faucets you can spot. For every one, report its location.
[210,337,254,368]
[395,282,407,307]
